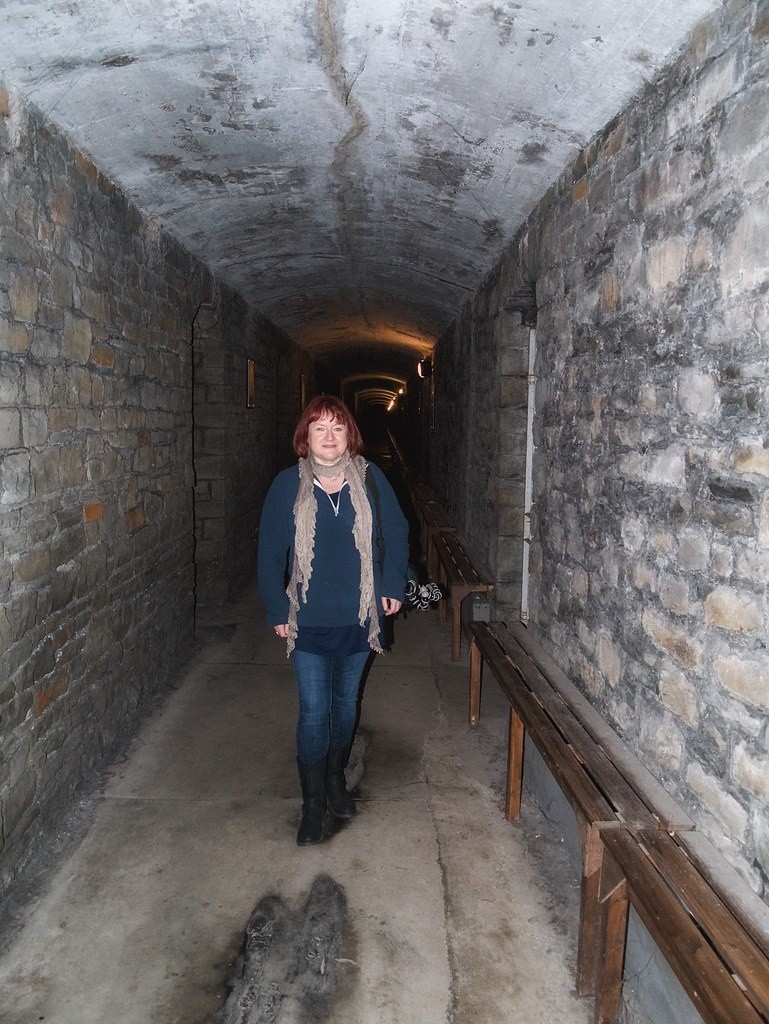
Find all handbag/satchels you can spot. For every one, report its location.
[394,562,443,620]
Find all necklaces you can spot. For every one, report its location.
[320,475,340,482]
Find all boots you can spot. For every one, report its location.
[296,753,329,845]
[328,750,356,818]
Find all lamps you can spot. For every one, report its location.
[417,355,433,378]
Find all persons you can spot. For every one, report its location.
[257,395,409,846]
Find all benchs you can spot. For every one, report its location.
[401,472,769,1024]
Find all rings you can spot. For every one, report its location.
[276,631,280,636]
